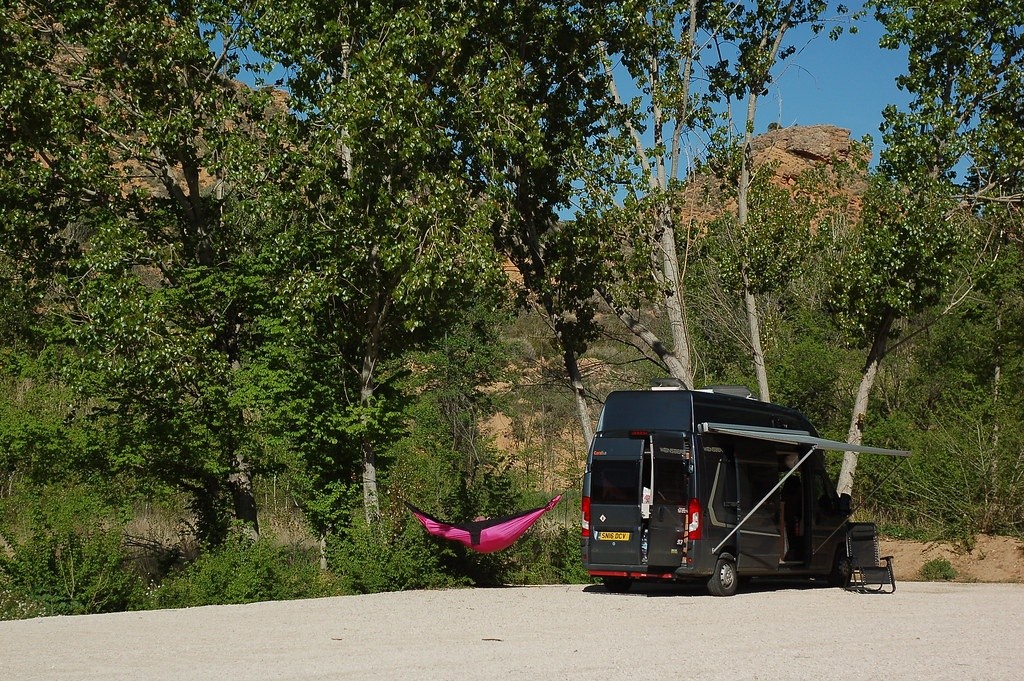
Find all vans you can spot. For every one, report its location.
[582,377,912,597]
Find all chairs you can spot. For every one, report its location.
[845,522,896,594]
[781,475,803,514]
